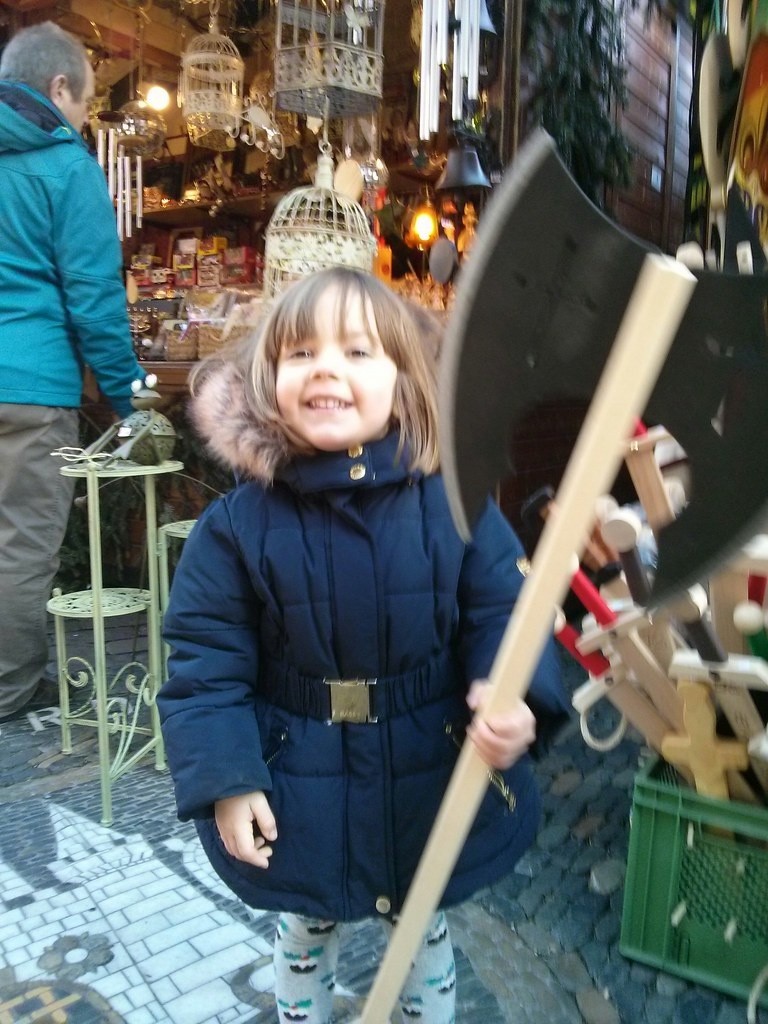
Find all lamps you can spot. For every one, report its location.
[407,200,439,249]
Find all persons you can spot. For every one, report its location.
[0,22,149,725]
[157,265,570,1024]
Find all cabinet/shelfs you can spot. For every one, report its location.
[118,116,313,331]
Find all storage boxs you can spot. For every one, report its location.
[616,747,768,1009]
[131,235,265,301]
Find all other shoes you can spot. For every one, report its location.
[0,675,72,724]
